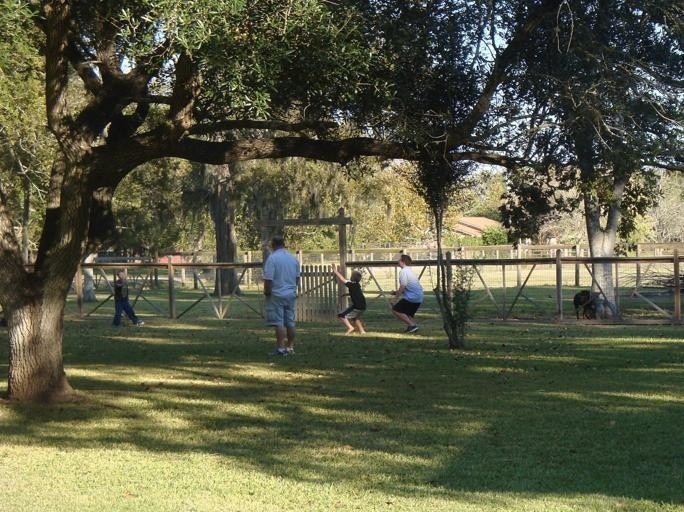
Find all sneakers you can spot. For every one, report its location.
[403,325,418,333]
[269,346,295,357]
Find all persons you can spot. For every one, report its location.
[113,272,145,327]
[264,237,301,356]
[391,255,424,332]
[332,266,366,334]
